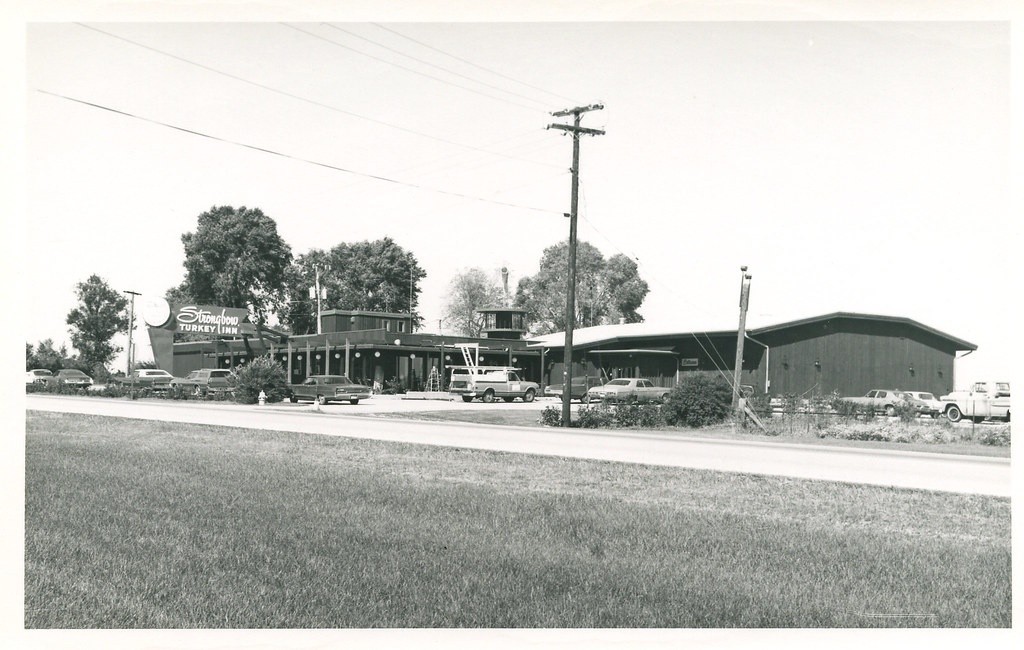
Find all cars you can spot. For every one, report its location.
[287,374,373,405]
[130,370,175,387]
[27,368,53,386]
[863,389,909,418]
[43,369,94,387]
[904,389,939,420]
[169,368,240,392]
[740,385,756,398]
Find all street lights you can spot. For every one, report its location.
[731,264,753,410]
[122,290,143,378]
[408,261,417,334]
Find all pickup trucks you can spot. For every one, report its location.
[448,370,539,403]
[543,376,608,404]
[588,378,674,404]
[939,380,1010,424]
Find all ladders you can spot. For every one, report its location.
[431,367,439,393]
[461,346,476,375]
